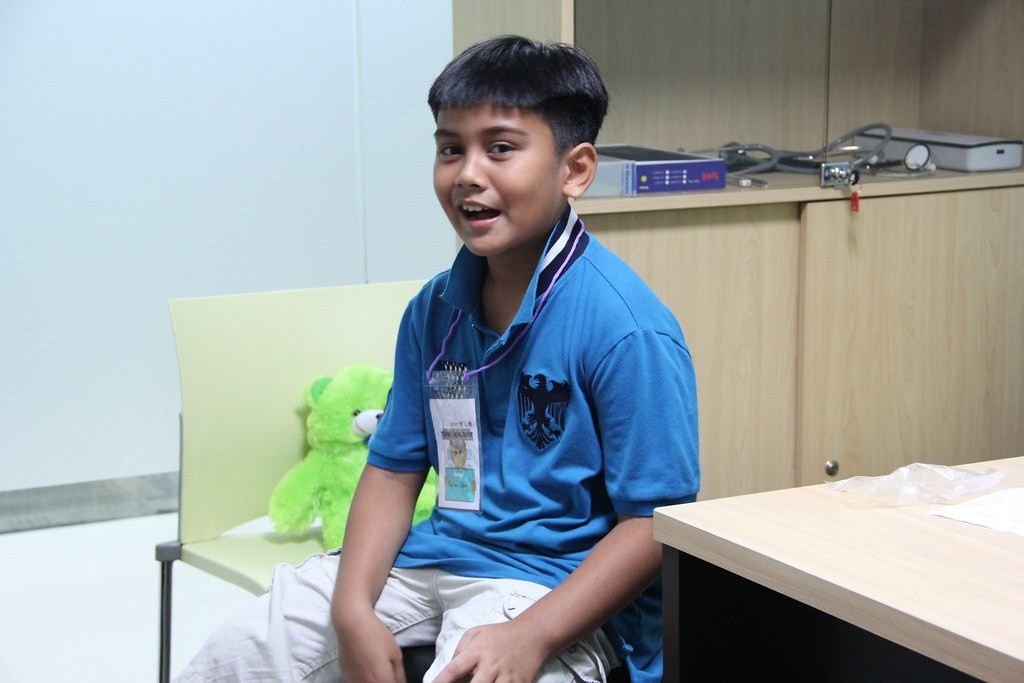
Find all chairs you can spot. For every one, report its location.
[157,280,427,683]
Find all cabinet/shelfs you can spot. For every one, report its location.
[454,0,1024,503]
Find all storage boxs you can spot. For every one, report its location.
[853,127,1024,174]
[581,154,637,198]
[597,142,727,198]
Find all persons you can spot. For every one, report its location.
[170,35,701,683]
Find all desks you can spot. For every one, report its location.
[650,454,1024,683]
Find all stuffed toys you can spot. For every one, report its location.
[268,364,440,552]
